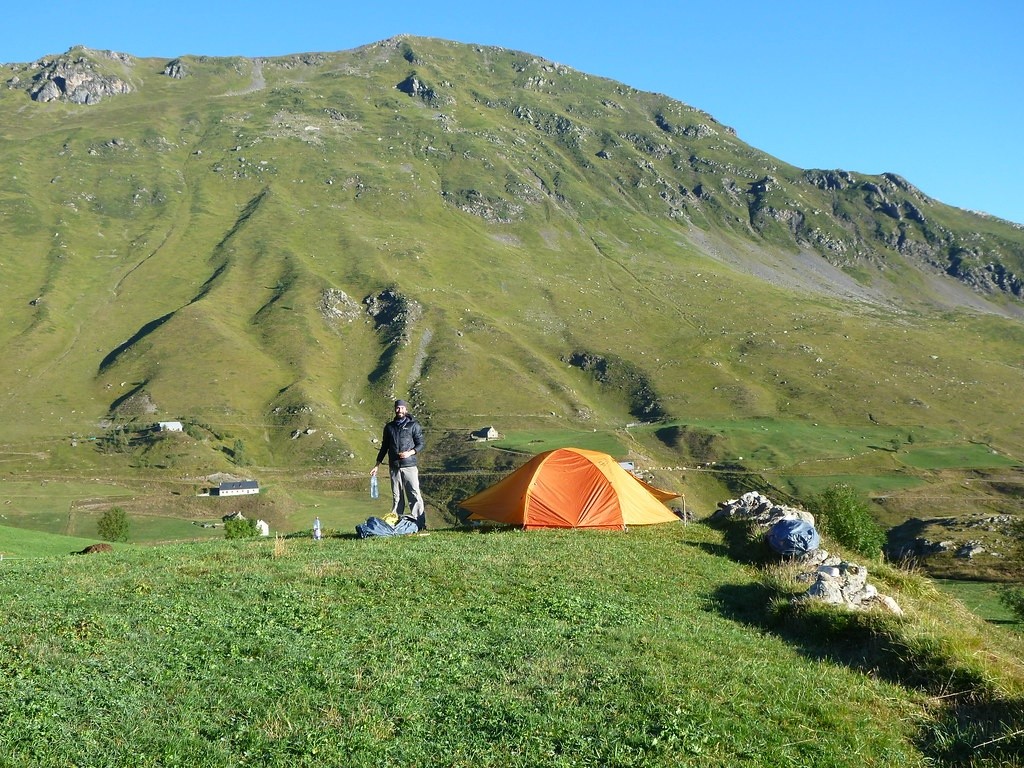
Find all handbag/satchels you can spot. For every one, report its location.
[356,516,418,536]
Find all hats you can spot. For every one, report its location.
[395,399,407,406]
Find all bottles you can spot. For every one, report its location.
[313,516,321,541]
[370,472,379,499]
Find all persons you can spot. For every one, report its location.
[369,400,428,531]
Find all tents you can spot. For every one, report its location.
[457,446,684,531]
